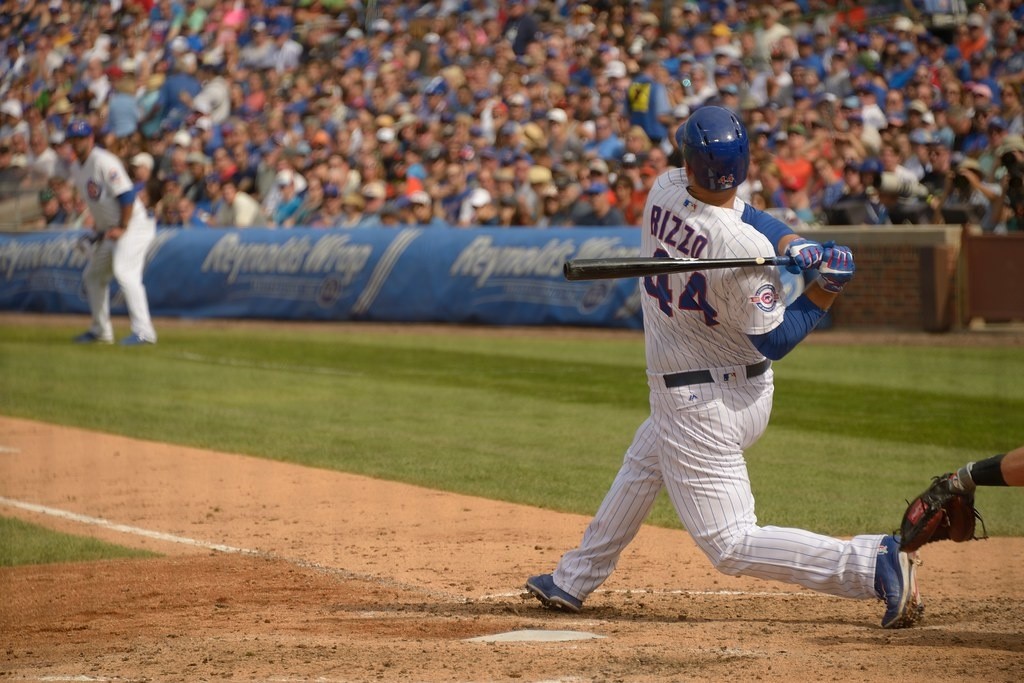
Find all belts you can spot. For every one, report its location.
[663,356,771,388]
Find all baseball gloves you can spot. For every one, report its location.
[893,462,988,553]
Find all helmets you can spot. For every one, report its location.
[67,119,92,137]
[675,105,750,191]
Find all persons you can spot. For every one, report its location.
[65,119,160,346]
[0,0,1023,234]
[523,107,922,632]
[900,444,1023,554]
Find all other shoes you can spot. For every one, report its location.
[75,332,116,344]
[120,335,146,344]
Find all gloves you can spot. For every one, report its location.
[784,237,824,274]
[816,239,856,294]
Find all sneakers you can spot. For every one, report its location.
[525,572,582,612]
[873,535,925,628]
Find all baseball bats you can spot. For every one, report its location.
[563,257,797,281]
